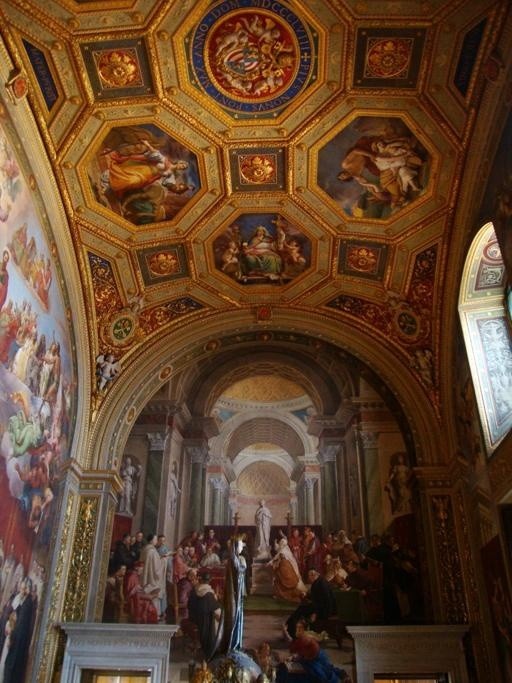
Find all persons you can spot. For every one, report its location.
[489,578,511,683]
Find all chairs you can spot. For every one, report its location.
[113,576,148,623]
[173,576,190,624]
[314,586,344,649]
[357,566,384,625]
